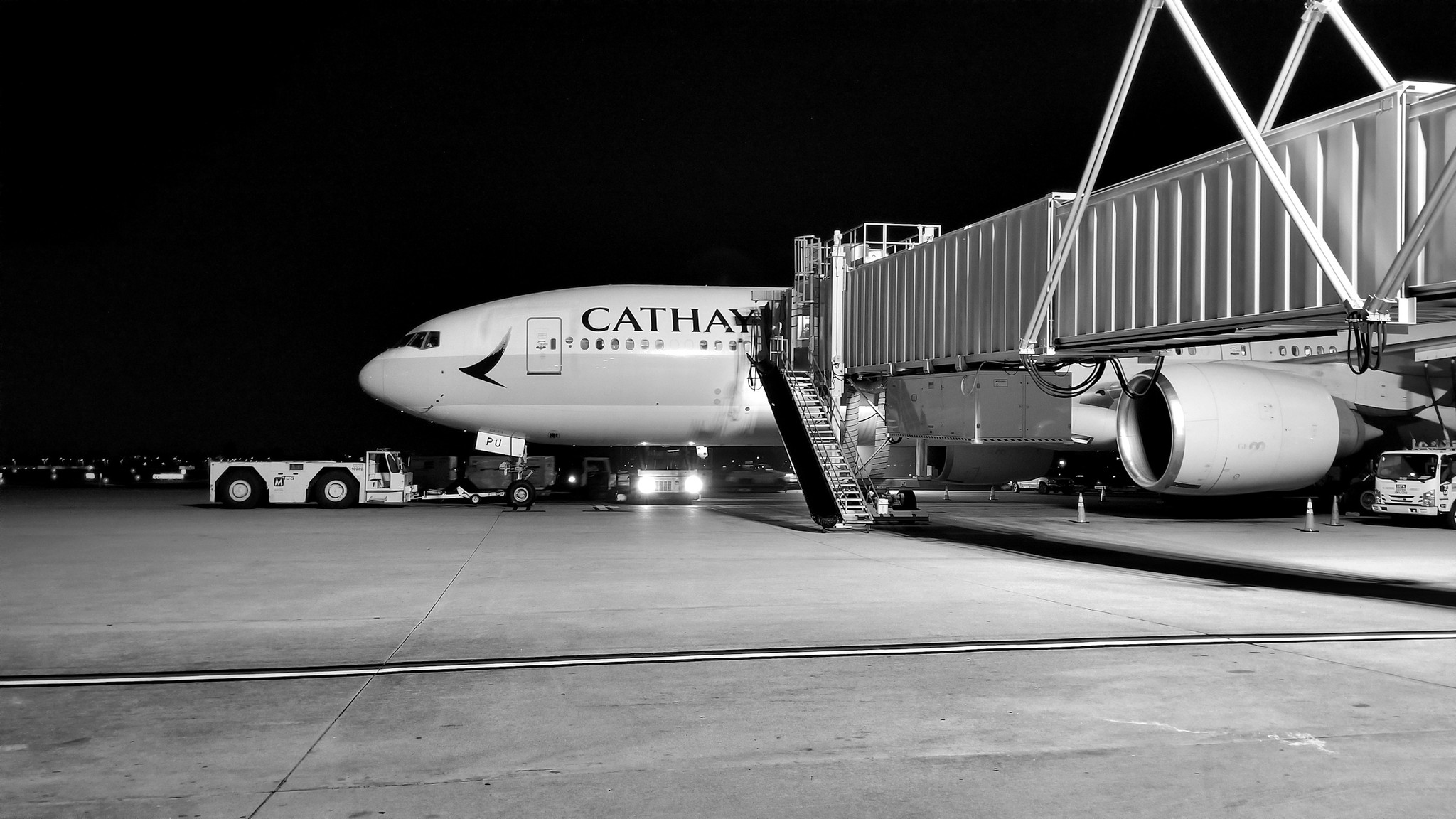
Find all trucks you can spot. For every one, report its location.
[1370,447,1456,529]
[627,449,699,506]
[210,448,423,509]
[576,457,630,503]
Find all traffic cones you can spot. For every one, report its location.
[1325,495,1344,527]
[988,486,998,500]
[1073,493,1090,523]
[942,485,950,500]
[1297,498,1319,533]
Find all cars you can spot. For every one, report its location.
[724,463,799,491]
[1008,477,1074,494]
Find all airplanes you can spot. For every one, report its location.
[356,222,1456,511]
